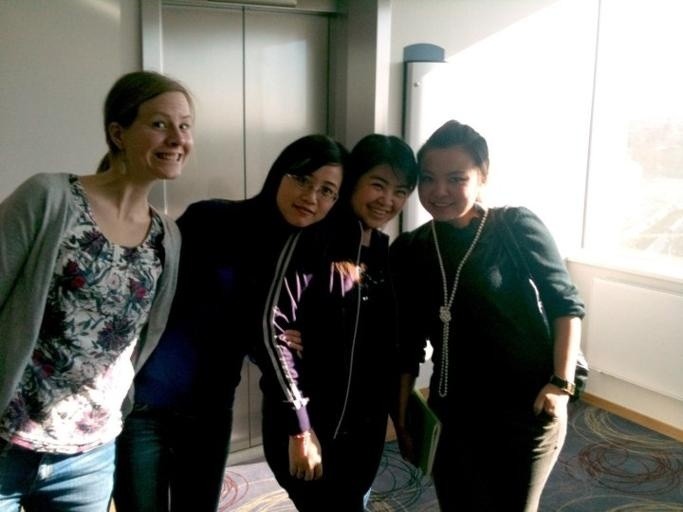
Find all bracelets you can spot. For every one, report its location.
[549,374,576,397]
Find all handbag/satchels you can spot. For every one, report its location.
[490,207,589,402]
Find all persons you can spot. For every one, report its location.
[257,133,417,512]
[113,134,349,512]
[393,120,587,512]
[0,70,195,512]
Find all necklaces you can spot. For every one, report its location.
[431,208,489,399]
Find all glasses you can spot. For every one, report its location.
[287,172,339,204]
